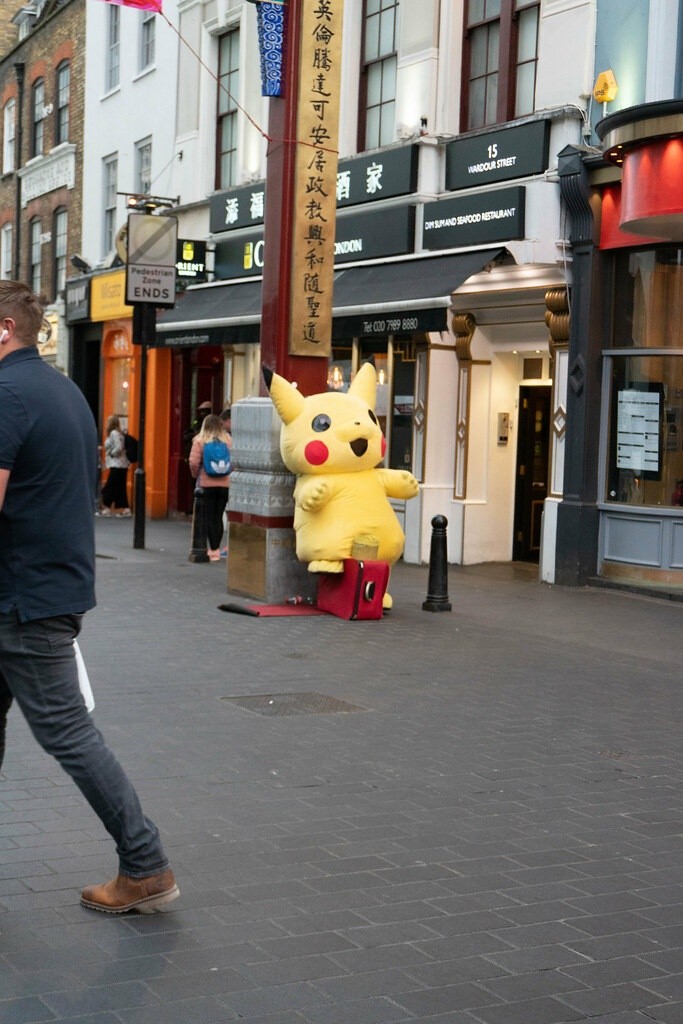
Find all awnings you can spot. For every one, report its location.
[151,245,509,351]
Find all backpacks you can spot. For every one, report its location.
[203,437,231,477]
[118,429,139,463]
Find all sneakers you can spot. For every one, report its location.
[94,510,112,518]
[116,509,132,518]
[81,867,181,913]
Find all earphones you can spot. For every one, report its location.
[0,330,8,342]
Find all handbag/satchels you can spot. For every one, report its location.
[73,638,96,713]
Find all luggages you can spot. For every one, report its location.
[316,559,389,622]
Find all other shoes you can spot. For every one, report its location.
[220,550,228,558]
[207,548,220,562]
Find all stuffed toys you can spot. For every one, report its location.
[259,360,420,618]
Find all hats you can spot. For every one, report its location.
[197,401,211,409]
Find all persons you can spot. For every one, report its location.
[0,275,182,919]
[99,398,233,561]
